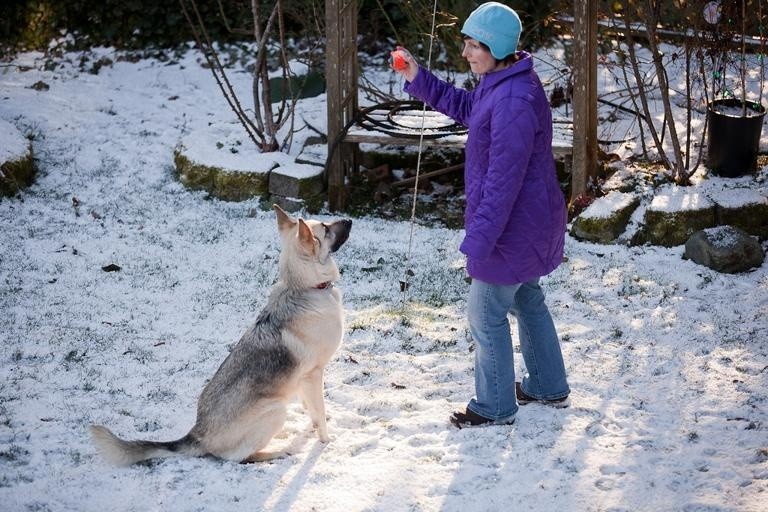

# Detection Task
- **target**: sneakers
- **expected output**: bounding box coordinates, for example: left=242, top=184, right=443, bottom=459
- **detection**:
left=448, top=407, right=516, bottom=431
left=513, top=380, right=571, bottom=411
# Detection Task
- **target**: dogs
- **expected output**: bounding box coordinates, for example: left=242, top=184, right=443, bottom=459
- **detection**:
left=85, top=203, right=353, bottom=469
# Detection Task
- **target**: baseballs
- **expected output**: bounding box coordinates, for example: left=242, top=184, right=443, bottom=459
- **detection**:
left=389, top=50, right=410, bottom=72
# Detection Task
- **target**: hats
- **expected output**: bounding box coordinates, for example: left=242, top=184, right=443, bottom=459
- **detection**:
left=457, top=1, right=523, bottom=61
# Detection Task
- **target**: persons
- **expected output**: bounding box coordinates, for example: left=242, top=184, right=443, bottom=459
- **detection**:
left=389, top=2, right=573, bottom=429
